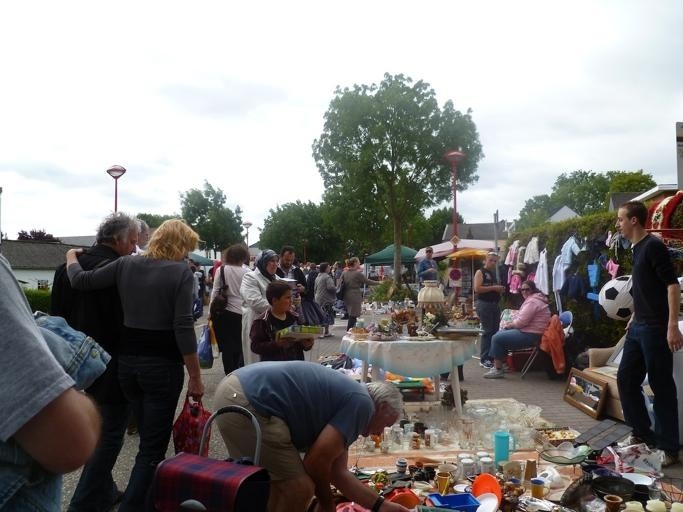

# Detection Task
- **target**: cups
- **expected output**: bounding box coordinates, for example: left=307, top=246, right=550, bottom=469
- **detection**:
left=366, top=441, right=376, bottom=452
left=508, top=479, right=526, bottom=494
left=495, top=431, right=516, bottom=470
left=461, top=458, right=478, bottom=480
left=435, top=472, right=451, bottom=495
left=384, top=427, right=397, bottom=448
left=394, top=428, right=403, bottom=445
left=480, top=457, right=496, bottom=474
left=425, top=430, right=437, bottom=449
left=455, top=418, right=478, bottom=450
left=438, top=464, right=457, bottom=485
left=404, top=424, right=415, bottom=435
left=352, top=327, right=364, bottom=341
left=531, top=480, right=550, bottom=500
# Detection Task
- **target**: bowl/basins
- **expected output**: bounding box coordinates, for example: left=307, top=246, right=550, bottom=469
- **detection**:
left=453, top=484, right=469, bottom=494
left=592, top=476, right=635, bottom=502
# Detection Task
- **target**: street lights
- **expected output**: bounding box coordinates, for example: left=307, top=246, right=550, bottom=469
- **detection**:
left=242, top=220, right=252, bottom=248
left=445, top=151, right=466, bottom=304
left=106, top=165, right=126, bottom=214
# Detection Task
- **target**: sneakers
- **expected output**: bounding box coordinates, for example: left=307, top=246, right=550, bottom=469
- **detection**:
left=483, top=368, right=505, bottom=379
left=652, top=450, right=673, bottom=466
left=618, top=436, right=645, bottom=448
left=490, top=362, right=512, bottom=373
left=479, top=360, right=492, bottom=368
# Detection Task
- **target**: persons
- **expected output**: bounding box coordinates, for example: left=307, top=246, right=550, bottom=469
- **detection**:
left=239, top=249, right=292, bottom=367
left=484, top=280, right=550, bottom=379
left=314, top=262, right=340, bottom=339
left=0, top=252, right=111, bottom=511
left=185, top=256, right=203, bottom=273
left=67, top=219, right=204, bottom=512
left=276, top=246, right=308, bottom=302
left=130, top=218, right=151, bottom=256
left=190, top=266, right=203, bottom=302
left=306, top=263, right=318, bottom=300
left=366, top=264, right=386, bottom=280
left=208, top=243, right=254, bottom=375
left=340, top=257, right=385, bottom=332
left=249, top=280, right=314, bottom=361
left=615, top=201, right=682, bottom=466
left=214, top=360, right=413, bottom=512
left=472, top=251, right=506, bottom=368
left=418, top=248, right=439, bottom=324
left=50, top=212, right=138, bottom=512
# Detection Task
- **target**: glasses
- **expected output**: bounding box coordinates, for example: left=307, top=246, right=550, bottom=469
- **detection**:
left=426, top=250, right=432, bottom=253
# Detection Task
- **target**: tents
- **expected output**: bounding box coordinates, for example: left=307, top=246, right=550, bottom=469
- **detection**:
left=364, top=243, right=419, bottom=264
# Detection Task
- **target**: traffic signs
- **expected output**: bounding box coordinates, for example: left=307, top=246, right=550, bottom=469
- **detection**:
left=448, top=267, right=463, bottom=288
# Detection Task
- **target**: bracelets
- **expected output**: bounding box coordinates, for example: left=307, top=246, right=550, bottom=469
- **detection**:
left=372, top=496, right=385, bottom=512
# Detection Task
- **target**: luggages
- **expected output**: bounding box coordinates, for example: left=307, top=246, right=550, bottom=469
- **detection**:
left=152, top=405, right=271, bottom=512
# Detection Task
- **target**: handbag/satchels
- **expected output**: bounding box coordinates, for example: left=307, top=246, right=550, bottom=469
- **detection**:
left=210, top=266, right=227, bottom=316
left=337, top=274, right=345, bottom=299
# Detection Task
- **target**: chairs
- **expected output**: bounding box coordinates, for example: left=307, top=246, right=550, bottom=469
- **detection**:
left=520, top=311, right=573, bottom=379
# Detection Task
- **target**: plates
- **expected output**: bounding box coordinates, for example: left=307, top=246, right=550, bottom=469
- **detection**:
left=472, top=473, right=502, bottom=509
left=536, top=442, right=593, bottom=464
left=619, top=473, right=653, bottom=486
left=475, top=493, right=499, bottom=512
